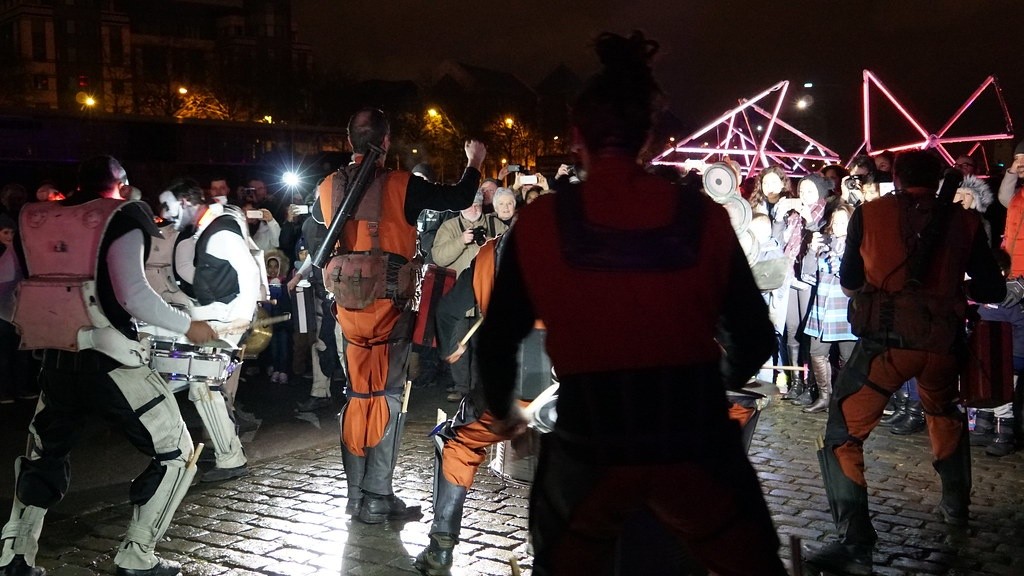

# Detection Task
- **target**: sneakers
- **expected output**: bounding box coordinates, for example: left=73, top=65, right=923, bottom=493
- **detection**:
left=938, top=496, right=970, bottom=526
left=414, top=544, right=453, bottom=576
left=359, top=494, right=421, bottom=523
left=299, top=395, right=331, bottom=412
left=344, top=497, right=363, bottom=516
left=801, top=539, right=873, bottom=576
left=115, top=556, right=182, bottom=576
left=201, top=463, right=248, bottom=482
left=0, top=558, right=46, bottom=576
left=448, top=391, right=464, bottom=401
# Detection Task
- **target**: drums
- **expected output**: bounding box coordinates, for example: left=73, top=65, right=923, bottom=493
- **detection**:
left=959, top=316, right=1017, bottom=411
left=146, top=337, right=233, bottom=389
left=701, top=161, right=762, bottom=269
left=411, top=264, right=457, bottom=349
left=492, top=418, right=537, bottom=490
left=534, top=394, right=558, bottom=431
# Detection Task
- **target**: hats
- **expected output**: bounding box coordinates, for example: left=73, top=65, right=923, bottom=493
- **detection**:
left=411, top=163, right=434, bottom=182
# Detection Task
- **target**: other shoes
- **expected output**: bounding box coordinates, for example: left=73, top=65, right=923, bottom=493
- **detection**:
left=333, top=369, right=345, bottom=381
left=235, top=412, right=258, bottom=433
left=244, top=367, right=258, bottom=377
left=279, top=373, right=288, bottom=385
left=304, top=371, right=313, bottom=379
left=266, top=367, right=275, bottom=376
left=271, top=371, right=280, bottom=383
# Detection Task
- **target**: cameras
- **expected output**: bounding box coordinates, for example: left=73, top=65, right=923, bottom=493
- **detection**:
left=243, top=188, right=256, bottom=199
left=469, top=226, right=487, bottom=247
left=566, top=165, right=576, bottom=174
left=846, top=174, right=867, bottom=190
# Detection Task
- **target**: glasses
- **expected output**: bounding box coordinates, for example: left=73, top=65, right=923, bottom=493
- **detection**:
left=953, top=163, right=974, bottom=169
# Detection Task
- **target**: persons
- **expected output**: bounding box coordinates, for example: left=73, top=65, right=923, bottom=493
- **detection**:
left=663, top=151, right=897, bottom=416
left=0, top=153, right=218, bottom=575
left=311, top=110, right=487, bottom=524
left=803, top=149, right=1008, bottom=576
left=0, top=177, right=65, bottom=404
left=414, top=230, right=556, bottom=576
left=475, top=29, right=790, bottom=576
left=411, top=162, right=578, bottom=402
left=144, top=176, right=345, bottom=483
left=879, top=141, right=1024, bottom=457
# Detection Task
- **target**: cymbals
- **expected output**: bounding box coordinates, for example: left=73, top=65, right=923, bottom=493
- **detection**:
left=741, top=378, right=781, bottom=396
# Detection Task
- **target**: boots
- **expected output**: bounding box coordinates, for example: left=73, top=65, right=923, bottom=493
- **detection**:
left=780, top=344, right=804, bottom=399
left=986, top=416, right=1017, bottom=457
left=878, top=397, right=908, bottom=426
left=891, top=401, right=925, bottom=435
left=803, top=354, right=833, bottom=414
left=968, top=410, right=995, bottom=447
left=792, top=358, right=819, bottom=405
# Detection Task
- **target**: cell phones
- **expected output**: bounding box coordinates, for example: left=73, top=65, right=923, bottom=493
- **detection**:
left=520, top=176, right=537, bottom=184
left=780, top=198, right=802, bottom=212
left=507, top=165, right=521, bottom=172
left=294, top=205, right=308, bottom=214
left=247, top=211, right=263, bottom=218
left=818, top=233, right=832, bottom=245
